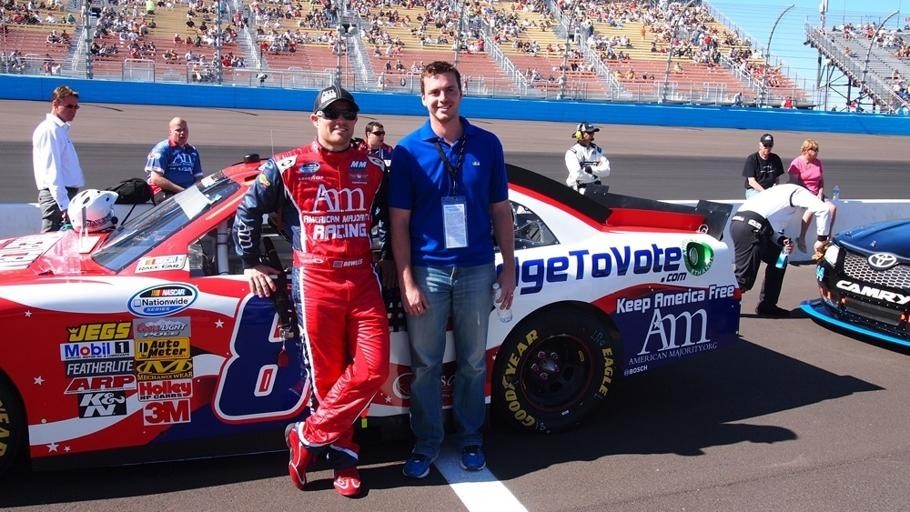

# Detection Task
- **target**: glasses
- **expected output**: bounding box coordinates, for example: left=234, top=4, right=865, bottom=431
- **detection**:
left=317, top=109, right=356, bottom=120
left=369, top=131, right=385, bottom=135
left=58, top=101, right=79, bottom=109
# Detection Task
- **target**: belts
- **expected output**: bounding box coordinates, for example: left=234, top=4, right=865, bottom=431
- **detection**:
left=731, top=215, right=761, bottom=229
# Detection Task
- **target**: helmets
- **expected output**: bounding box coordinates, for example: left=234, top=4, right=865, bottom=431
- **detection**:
left=575, top=122, right=600, bottom=140
left=68, top=189, right=118, bottom=233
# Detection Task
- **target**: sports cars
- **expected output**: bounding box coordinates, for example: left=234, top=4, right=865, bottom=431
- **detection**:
left=1, top=149, right=742, bottom=495
left=799, top=218, right=910, bottom=348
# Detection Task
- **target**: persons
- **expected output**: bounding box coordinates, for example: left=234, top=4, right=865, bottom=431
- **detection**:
left=359, top=121, right=393, bottom=160
left=742, top=133, right=784, bottom=200
left=787, top=139, right=837, bottom=254
left=386, top=61, right=516, bottom=479
left=144, top=117, right=205, bottom=203
left=32, top=86, right=86, bottom=233
left=231, top=84, right=397, bottom=496
left=1, top=0, right=909, bottom=117
left=565, top=122, right=610, bottom=195
left=729, top=179, right=831, bottom=318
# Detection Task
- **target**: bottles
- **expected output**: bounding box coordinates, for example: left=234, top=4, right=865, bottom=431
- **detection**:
left=775, top=249, right=791, bottom=269
left=832, top=184, right=840, bottom=201
left=490, top=280, right=513, bottom=323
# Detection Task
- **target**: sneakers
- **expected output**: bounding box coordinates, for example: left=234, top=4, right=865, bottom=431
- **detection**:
left=285, top=423, right=312, bottom=489
left=403, top=451, right=439, bottom=477
left=459, top=444, right=486, bottom=471
left=334, top=464, right=363, bottom=495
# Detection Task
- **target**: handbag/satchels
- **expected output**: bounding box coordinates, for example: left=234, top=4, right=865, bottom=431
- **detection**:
left=106, top=178, right=155, bottom=204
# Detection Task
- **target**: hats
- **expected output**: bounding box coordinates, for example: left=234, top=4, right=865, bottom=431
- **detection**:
left=314, top=87, right=359, bottom=114
left=761, top=134, right=773, bottom=146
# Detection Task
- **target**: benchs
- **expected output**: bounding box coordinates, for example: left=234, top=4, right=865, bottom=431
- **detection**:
left=1, top=0, right=814, bottom=107
left=804, top=24, right=910, bottom=114
